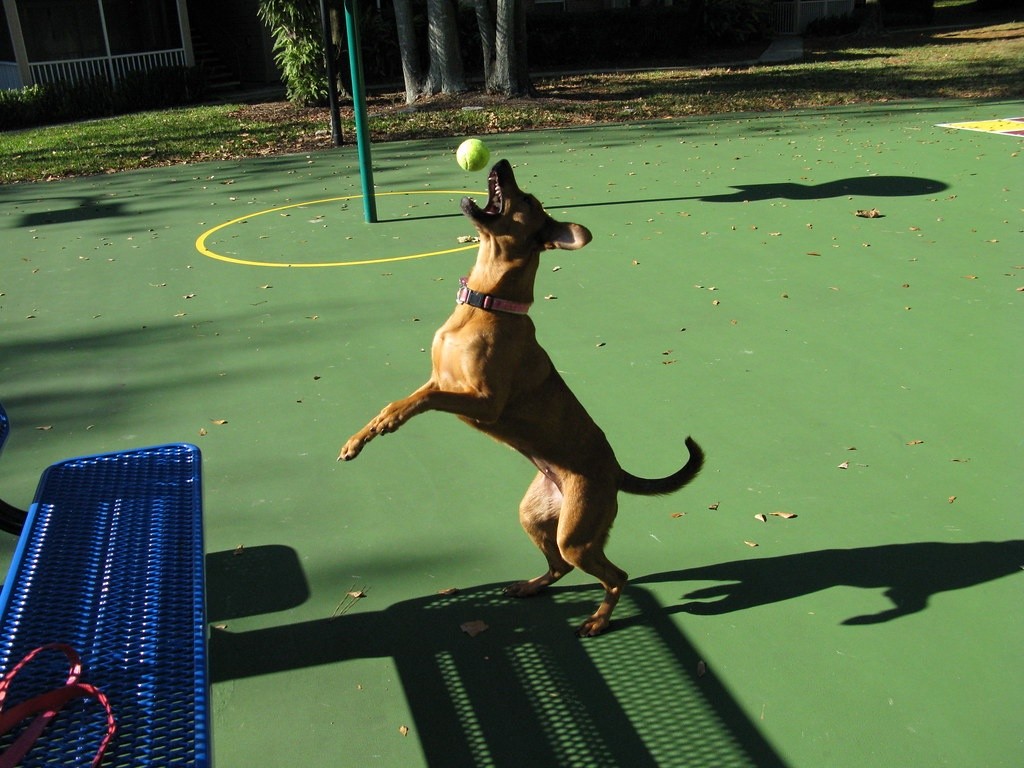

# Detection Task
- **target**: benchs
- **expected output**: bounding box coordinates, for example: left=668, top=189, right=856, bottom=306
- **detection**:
left=0, top=406, right=212, bottom=768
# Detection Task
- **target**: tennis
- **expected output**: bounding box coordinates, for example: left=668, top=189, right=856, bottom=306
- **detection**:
left=456, top=137, right=490, bottom=172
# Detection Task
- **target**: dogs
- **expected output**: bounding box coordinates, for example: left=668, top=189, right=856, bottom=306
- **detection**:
left=337, top=157, right=706, bottom=638
